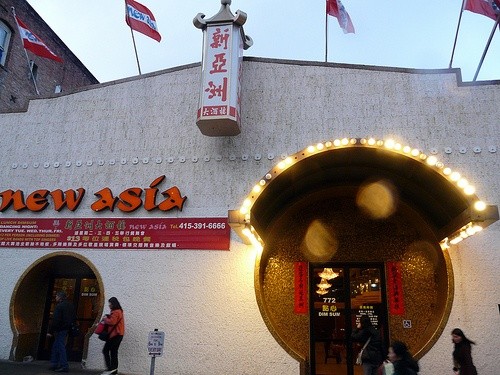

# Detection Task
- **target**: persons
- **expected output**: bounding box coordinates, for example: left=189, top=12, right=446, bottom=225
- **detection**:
left=101, top=297, right=124, bottom=375
left=387, top=341, right=419, bottom=375
left=351, top=315, right=383, bottom=375
left=450, top=328, right=477, bottom=375
left=46, top=290, right=72, bottom=372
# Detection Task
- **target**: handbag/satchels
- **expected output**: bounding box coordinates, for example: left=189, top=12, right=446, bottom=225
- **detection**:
left=98, top=326, right=109, bottom=341
left=355, top=349, right=364, bottom=366
left=69, top=320, right=80, bottom=337
left=382, top=360, right=395, bottom=375
left=95, top=321, right=105, bottom=335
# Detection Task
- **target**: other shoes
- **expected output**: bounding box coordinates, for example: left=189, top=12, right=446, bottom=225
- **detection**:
left=100, top=368, right=118, bottom=375
left=48, top=363, right=69, bottom=373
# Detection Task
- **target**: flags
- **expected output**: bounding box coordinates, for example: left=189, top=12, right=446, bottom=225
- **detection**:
left=124, top=0, right=161, bottom=43
left=326, top=0, right=356, bottom=34
left=14, top=14, right=64, bottom=62
left=463, top=0, right=500, bottom=30
